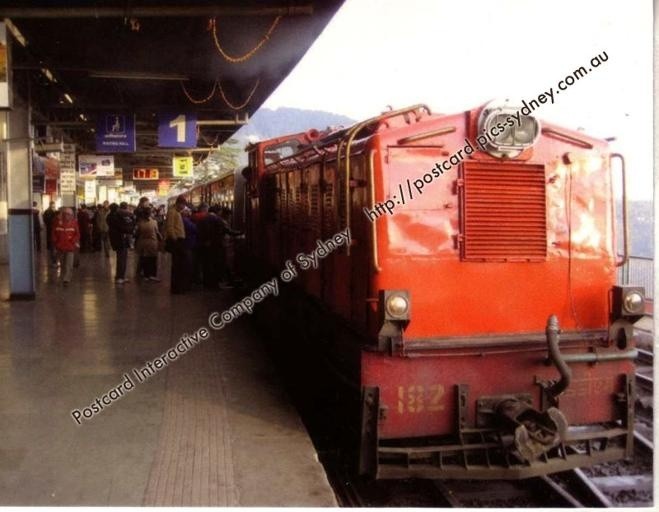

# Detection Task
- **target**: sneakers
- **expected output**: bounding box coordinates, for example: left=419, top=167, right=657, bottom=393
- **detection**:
left=124, top=277, right=131, bottom=282
left=115, top=279, right=123, bottom=285
left=144, top=276, right=161, bottom=282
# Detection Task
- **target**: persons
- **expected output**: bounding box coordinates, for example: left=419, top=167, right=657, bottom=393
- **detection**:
left=242, top=166, right=252, bottom=180
left=33, top=196, right=243, bottom=294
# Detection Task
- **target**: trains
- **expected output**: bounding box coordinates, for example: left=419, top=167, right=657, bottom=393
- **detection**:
left=166, top=94, right=646, bottom=483
